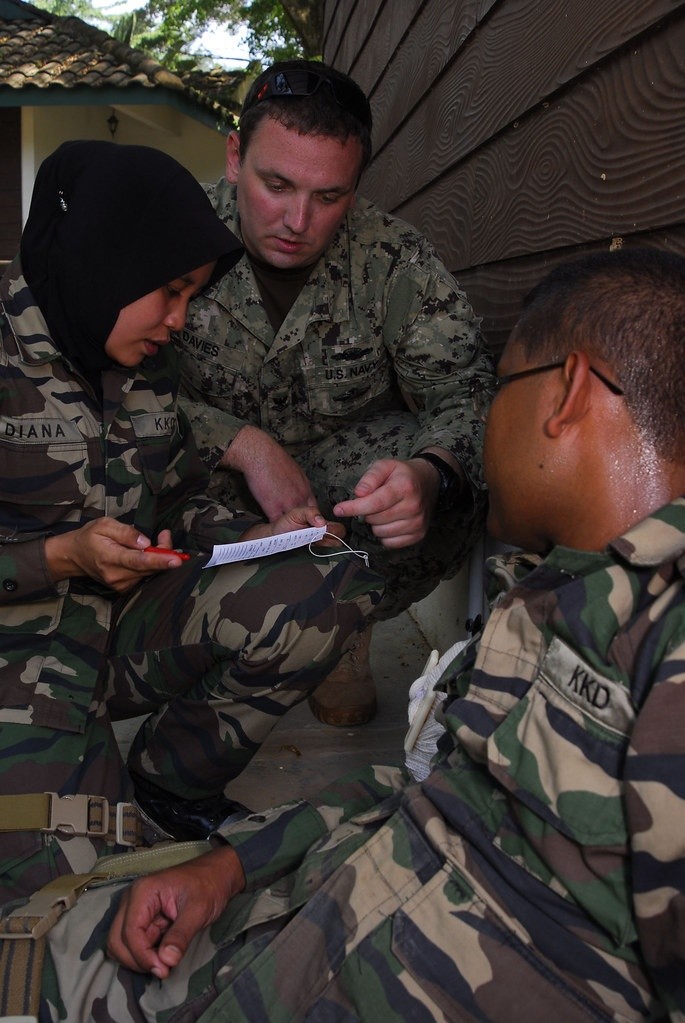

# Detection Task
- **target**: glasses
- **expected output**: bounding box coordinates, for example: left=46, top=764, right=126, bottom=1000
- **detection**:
left=468, top=361, right=624, bottom=424
left=239, top=68, right=372, bottom=131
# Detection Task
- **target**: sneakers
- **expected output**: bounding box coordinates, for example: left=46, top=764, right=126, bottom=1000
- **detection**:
left=308, top=622, right=379, bottom=725
left=125, top=764, right=259, bottom=843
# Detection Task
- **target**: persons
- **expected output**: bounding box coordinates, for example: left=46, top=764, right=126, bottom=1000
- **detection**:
left=130, top=59, right=505, bottom=628
left=2, top=131, right=380, bottom=899
left=29, top=249, right=684, bottom=1023
